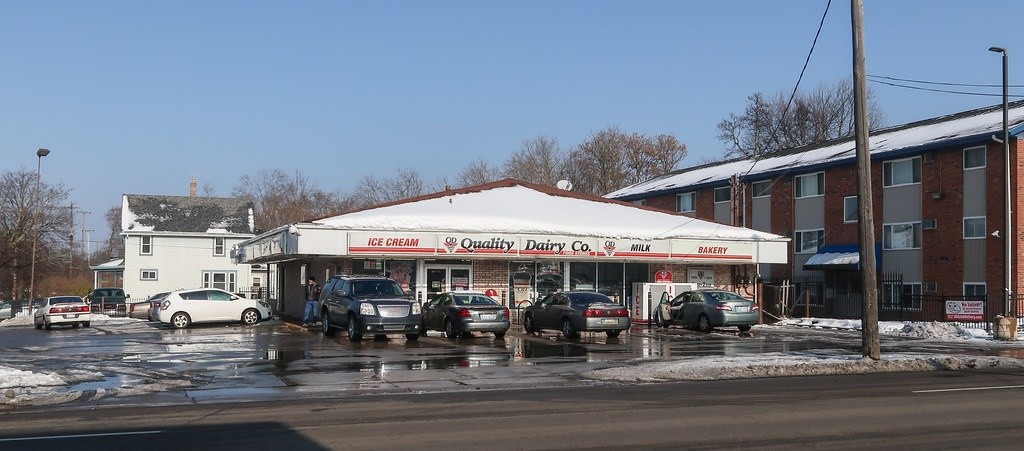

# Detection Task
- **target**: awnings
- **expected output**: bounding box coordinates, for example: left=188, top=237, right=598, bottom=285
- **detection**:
left=803, top=243, right=882, bottom=273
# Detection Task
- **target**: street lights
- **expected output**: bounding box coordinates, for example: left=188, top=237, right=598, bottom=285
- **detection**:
left=988, top=45, right=1017, bottom=342
left=28, top=148, right=51, bottom=315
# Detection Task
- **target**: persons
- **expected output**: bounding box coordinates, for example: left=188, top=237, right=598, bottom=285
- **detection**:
left=303, top=276, right=321, bottom=327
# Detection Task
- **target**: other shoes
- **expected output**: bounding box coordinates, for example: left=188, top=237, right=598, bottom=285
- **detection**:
left=302, top=324, right=308, bottom=327
left=312, top=323, right=316, bottom=327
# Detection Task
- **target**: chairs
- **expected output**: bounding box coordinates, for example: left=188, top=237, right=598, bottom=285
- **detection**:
left=456, top=298, right=464, bottom=305
left=472, top=300, right=480, bottom=304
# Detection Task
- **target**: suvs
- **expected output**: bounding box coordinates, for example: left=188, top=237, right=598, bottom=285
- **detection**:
left=89, top=288, right=130, bottom=316
left=318, top=273, right=424, bottom=341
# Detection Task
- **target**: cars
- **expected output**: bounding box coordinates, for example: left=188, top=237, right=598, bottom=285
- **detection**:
left=129, top=293, right=157, bottom=322
left=652, top=288, right=759, bottom=333
left=148, top=289, right=274, bottom=328
left=33, top=296, right=91, bottom=330
left=0, top=298, right=44, bottom=319
left=420, top=292, right=510, bottom=339
left=520, top=291, right=632, bottom=338
